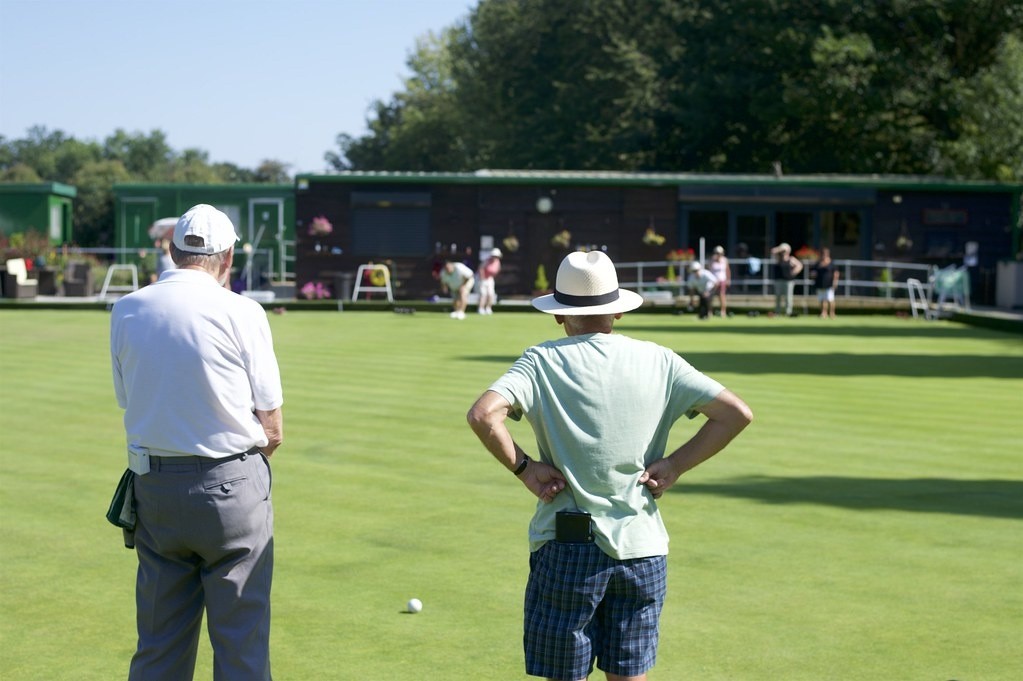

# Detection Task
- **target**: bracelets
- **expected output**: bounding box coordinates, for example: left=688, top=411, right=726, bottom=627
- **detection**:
left=512, top=453, right=530, bottom=475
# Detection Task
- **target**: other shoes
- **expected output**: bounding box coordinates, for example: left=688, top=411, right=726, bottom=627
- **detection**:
left=478, top=308, right=495, bottom=314
left=450, top=310, right=466, bottom=320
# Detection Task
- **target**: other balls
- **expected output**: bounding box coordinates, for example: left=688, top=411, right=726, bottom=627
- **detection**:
left=408, top=598, right=422, bottom=614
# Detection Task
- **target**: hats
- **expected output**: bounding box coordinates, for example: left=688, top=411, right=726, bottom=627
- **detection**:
left=491, top=248, right=502, bottom=257
left=714, top=245, right=725, bottom=254
left=691, top=261, right=701, bottom=272
left=172, top=204, right=241, bottom=256
left=532, top=250, right=643, bottom=315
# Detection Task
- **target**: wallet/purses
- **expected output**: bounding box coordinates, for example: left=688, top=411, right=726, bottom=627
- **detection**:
left=555, top=511, right=592, bottom=543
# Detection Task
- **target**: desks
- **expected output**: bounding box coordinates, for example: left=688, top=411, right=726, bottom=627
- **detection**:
left=39, top=271, right=59, bottom=295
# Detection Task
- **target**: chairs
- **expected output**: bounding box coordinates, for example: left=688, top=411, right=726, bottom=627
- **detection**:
left=4, top=256, right=39, bottom=300
left=61, top=259, right=92, bottom=298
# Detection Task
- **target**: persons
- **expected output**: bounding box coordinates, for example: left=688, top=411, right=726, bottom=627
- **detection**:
left=770, top=243, right=803, bottom=317
left=429, top=248, right=503, bottom=319
left=466, top=251, right=752, bottom=680
left=809, top=247, right=839, bottom=317
left=685, top=242, right=751, bottom=320
left=107, top=203, right=284, bottom=680
